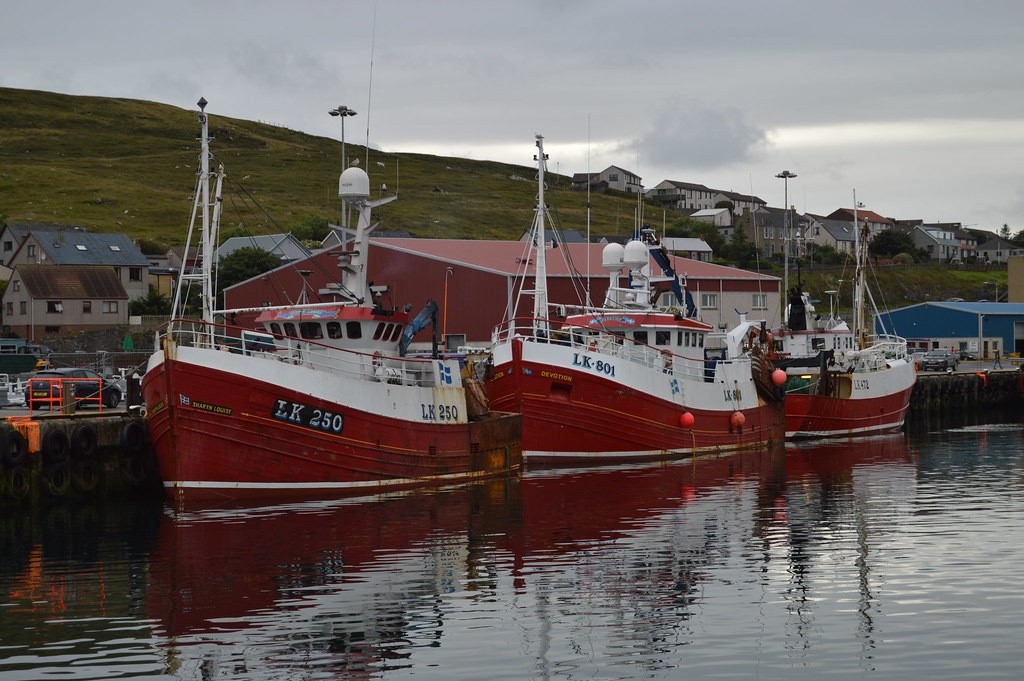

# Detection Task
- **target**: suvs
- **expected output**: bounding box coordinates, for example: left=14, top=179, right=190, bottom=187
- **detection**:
left=19, top=345, right=53, bottom=362
left=907, top=348, right=960, bottom=372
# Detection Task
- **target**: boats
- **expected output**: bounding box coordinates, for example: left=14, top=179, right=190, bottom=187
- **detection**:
left=760, top=188, right=919, bottom=440
left=140, top=97, right=522, bottom=512
left=491, top=448, right=776, bottom=589
left=137, top=486, right=472, bottom=676
left=483, top=133, right=789, bottom=473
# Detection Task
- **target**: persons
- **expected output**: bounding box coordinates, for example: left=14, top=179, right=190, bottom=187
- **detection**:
left=993, top=349, right=1003, bottom=369
left=921, top=349, right=927, bottom=370
left=952, top=347, right=960, bottom=364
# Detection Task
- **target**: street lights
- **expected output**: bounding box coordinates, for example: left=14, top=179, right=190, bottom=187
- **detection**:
left=328, top=105, right=359, bottom=288
left=775, top=170, right=798, bottom=330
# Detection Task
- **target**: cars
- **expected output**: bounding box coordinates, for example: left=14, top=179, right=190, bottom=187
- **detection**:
left=960, top=352, right=977, bottom=361
left=24, top=368, right=121, bottom=410
